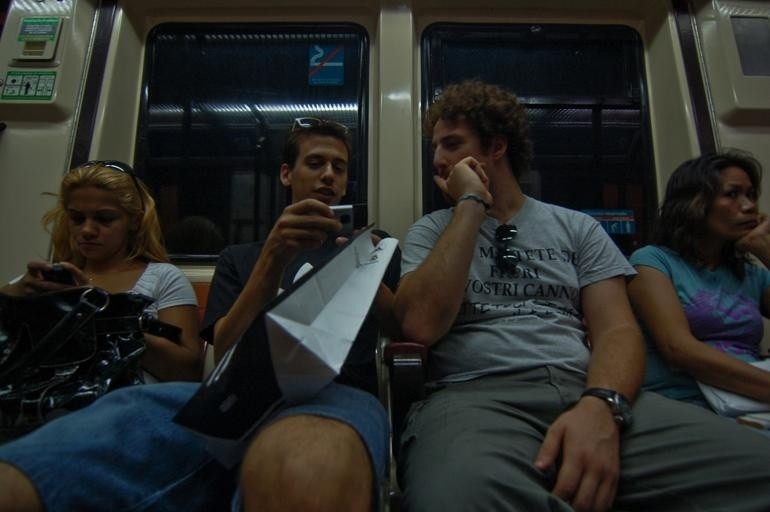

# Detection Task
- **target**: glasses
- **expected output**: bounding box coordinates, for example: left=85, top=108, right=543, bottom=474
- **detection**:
left=75, top=159, right=146, bottom=214
left=494, top=222, right=520, bottom=278
left=292, top=116, right=348, bottom=137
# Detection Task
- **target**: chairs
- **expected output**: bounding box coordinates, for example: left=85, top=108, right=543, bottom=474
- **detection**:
left=137, top=274, right=426, bottom=511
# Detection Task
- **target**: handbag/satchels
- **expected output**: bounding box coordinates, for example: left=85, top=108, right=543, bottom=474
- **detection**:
left=172, top=224, right=400, bottom=449
left=0, top=283, right=156, bottom=446
left=694, top=358, right=770, bottom=418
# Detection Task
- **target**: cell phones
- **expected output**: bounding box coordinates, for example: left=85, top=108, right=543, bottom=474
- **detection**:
left=329, top=205, right=354, bottom=233
left=41, top=264, right=79, bottom=286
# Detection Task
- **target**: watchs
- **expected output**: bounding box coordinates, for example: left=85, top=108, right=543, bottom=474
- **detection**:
left=580, top=387, right=633, bottom=430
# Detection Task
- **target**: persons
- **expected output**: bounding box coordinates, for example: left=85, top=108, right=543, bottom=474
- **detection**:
left=393, top=79, right=769, bottom=512
left=0, top=116, right=403, bottom=512
left=626, top=146, right=770, bottom=420
left=0, top=159, right=203, bottom=439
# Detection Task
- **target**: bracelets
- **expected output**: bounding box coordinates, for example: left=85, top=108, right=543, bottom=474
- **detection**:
left=457, top=193, right=490, bottom=212
